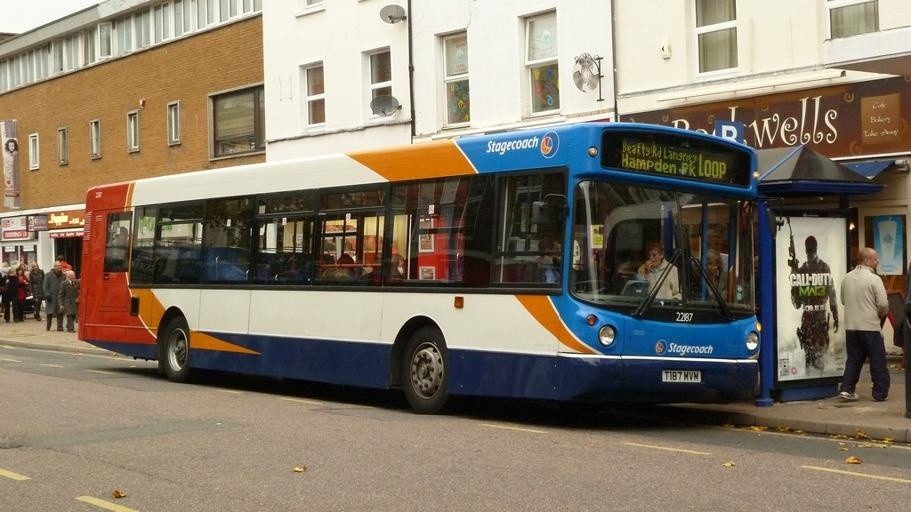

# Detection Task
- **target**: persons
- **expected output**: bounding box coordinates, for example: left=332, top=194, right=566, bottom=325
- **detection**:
left=532, top=236, right=564, bottom=284
left=4, top=139, right=18, bottom=155
left=0, top=254, right=80, bottom=333
left=637, top=239, right=681, bottom=301
left=111, top=227, right=128, bottom=258
left=841, top=247, right=891, bottom=402
left=788, top=235, right=839, bottom=378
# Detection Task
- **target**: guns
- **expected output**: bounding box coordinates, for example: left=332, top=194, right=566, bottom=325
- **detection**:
left=783, top=217, right=800, bottom=280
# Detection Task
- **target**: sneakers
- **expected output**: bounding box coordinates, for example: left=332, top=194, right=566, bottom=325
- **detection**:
left=839, top=390, right=862, bottom=402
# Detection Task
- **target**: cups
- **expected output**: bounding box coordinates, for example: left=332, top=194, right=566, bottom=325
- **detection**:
left=878, top=218, right=897, bottom=269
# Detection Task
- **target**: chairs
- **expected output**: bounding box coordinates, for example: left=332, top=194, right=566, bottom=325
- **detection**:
left=166, top=244, right=335, bottom=285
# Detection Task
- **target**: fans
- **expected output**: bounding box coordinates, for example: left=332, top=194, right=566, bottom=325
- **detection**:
left=573, top=54, right=604, bottom=101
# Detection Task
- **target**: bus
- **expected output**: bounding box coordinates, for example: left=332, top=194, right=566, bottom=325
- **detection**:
left=76, top=121, right=765, bottom=414
